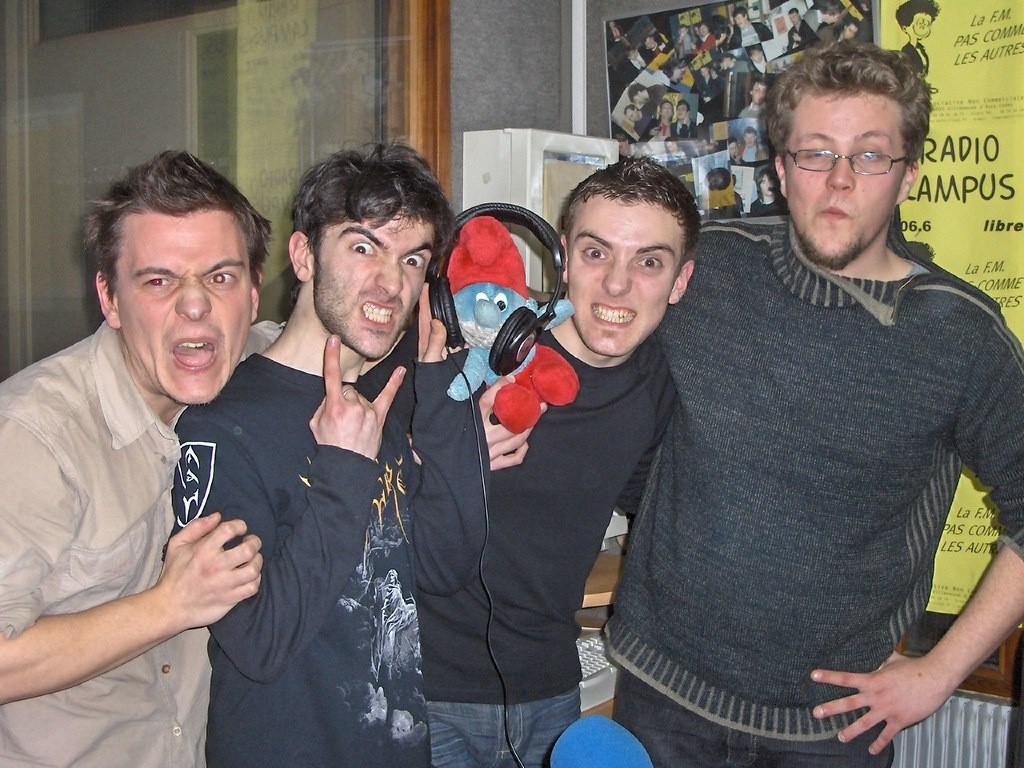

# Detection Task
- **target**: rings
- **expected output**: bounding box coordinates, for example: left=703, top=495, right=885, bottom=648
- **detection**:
left=343, top=388, right=358, bottom=398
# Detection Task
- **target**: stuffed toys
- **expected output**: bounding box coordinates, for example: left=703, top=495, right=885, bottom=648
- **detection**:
left=442, top=217, right=581, bottom=433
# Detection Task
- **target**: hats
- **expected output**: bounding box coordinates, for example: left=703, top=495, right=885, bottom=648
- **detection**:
left=754, top=164, right=776, bottom=180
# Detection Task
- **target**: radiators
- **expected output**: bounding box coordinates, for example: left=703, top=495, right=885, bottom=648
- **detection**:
left=891, top=688, right=1024, bottom=768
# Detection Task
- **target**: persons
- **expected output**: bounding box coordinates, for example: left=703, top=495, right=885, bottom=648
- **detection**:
left=1, top=154, right=287, bottom=768
left=604, top=47, right=1024, bottom=766
left=606, top=0, right=869, bottom=216
left=419, top=160, right=701, bottom=767
left=163, top=151, right=489, bottom=767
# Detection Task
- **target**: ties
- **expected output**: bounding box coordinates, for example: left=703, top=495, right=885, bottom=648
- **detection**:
left=662, top=123, right=668, bottom=135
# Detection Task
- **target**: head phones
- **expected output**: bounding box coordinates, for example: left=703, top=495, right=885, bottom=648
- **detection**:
left=428, top=201, right=565, bottom=377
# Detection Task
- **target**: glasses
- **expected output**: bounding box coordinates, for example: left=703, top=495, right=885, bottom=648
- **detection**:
left=786, top=146, right=908, bottom=176
